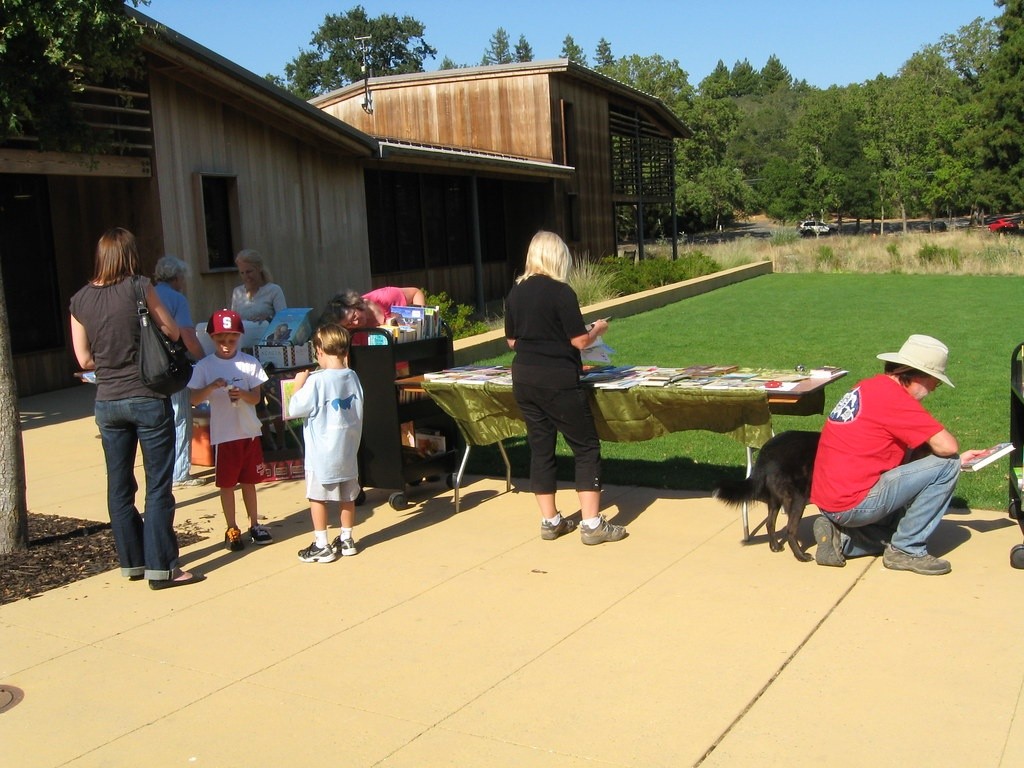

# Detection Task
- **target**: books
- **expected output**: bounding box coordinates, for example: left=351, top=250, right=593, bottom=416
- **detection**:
left=581, top=364, right=812, bottom=391
left=960, top=441, right=1016, bottom=473
left=396, top=359, right=448, bottom=458
left=281, top=379, right=304, bottom=421
left=368, top=304, right=440, bottom=346
left=424, top=363, right=513, bottom=387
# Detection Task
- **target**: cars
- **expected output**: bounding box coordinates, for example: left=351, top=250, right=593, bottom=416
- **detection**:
left=798, top=220, right=838, bottom=237
left=984, top=212, right=1024, bottom=234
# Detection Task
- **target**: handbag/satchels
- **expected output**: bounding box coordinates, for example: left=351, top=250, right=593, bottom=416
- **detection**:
left=132, top=274, right=195, bottom=395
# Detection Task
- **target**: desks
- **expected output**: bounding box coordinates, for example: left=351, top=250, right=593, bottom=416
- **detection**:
left=255, top=363, right=319, bottom=456
left=421, top=366, right=849, bottom=545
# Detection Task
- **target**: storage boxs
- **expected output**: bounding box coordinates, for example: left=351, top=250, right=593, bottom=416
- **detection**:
left=191, top=307, right=315, bottom=481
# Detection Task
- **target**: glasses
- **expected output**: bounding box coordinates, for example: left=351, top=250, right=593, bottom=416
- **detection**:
left=344, top=310, right=357, bottom=329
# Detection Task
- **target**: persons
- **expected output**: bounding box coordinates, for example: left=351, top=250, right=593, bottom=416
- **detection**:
left=503, top=231, right=628, bottom=546
left=319, top=285, right=425, bottom=346
left=69, top=226, right=206, bottom=591
left=186, top=307, right=273, bottom=551
left=809, top=334, right=987, bottom=574
left=288, top=322, right=365, bottom=563
left=147, top=254, right=206, bottom=488
left=230, top=250, right=290, bottom=321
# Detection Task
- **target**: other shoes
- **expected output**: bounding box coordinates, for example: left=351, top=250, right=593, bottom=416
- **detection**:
left=172, top=478, right=207, bottom=490
left=148, top=571, right=203, bottom=589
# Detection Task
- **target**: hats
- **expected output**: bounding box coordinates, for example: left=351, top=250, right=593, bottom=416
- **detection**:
left=206, top=307, right=246, bottom=337
left=875, top=334, right=955, bottom=391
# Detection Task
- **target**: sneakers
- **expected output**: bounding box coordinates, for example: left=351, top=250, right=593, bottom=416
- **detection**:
left=329, top=536, right=358, bottom=555
left=297, top=542, right=336, bottom=563
left=580, top=515, right=626, bottom=545
left=882, top=544, right=952, bottom=575
left=812, top=516, right=848, bottom=567
left=251, top=525, right=274, bottom=545
left=223, top=529, right=245, bottom=551
left=541, top=516, right=575, bottom=541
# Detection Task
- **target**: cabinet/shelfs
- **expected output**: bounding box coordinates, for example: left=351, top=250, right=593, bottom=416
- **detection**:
left=1008, top=343, right=1024, bottom=568
left=348, top=323, right=464, bottom=511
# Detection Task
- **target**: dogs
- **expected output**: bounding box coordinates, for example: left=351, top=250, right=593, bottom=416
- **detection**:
left=717, top=430, right=932, bottom=563
